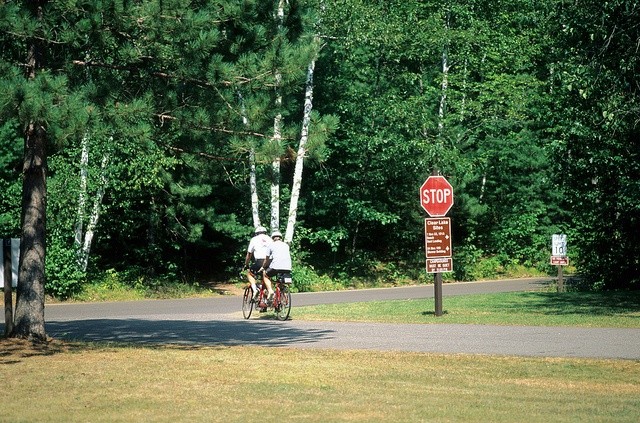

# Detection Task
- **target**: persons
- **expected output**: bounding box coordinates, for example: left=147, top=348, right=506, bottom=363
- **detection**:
left=257, top=230, right=293, bottom=306
left=242, top=226, right=273, bottom=300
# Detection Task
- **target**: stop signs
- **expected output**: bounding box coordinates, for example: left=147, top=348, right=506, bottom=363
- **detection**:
left=420, top=175, right=454, bottom=217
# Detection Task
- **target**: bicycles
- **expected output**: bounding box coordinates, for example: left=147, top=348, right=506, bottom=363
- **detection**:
left=242, top=262, right=291, bottom=321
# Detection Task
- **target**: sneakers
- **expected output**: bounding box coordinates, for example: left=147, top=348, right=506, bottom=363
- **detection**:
left=252, top=290, right=258, bottom=299
left=267, top=290, right=274, bottom=300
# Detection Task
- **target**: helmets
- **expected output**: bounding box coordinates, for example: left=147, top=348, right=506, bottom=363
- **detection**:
left=255, top=226, right=267, bottom=234
left=271, top=232, right=282, bottom=239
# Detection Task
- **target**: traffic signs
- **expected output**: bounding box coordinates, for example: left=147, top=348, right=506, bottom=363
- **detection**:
left=552, top=234, right=567, bottom=255
left=425, top=217, right=452, bottom=258
left=426, top=257, right=453, bottom=273
left=550, top=256, right=569, bottom=265
